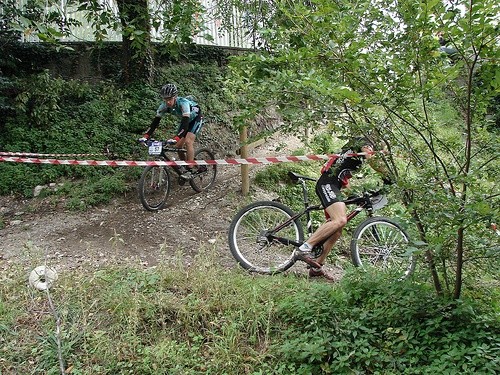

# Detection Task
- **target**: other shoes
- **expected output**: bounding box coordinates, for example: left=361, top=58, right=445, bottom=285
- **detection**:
left=180, top=172, right=193, bottom=179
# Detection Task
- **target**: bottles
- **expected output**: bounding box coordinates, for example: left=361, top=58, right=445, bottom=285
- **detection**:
left=171, top=157, right=184, bottom=171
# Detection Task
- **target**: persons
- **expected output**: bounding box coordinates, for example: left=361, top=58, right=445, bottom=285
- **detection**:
left=295, top=130, right=397, bottom=282
left=142, top=84, right=204, bottom=178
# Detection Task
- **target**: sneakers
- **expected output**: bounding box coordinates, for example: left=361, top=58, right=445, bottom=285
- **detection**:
left=294, top=247, right=321, bottom=269
left=309, top=268, right=334, bottom=280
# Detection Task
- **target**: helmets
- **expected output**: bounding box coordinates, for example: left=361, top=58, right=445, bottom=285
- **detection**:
left=160, top=84, right=177, bottom=98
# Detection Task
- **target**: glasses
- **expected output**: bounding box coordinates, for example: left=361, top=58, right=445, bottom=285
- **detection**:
left=162, top=96, right=174, bottom=102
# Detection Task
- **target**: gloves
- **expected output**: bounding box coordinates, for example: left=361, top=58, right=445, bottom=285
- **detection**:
left=167, top=139, right=177, bottom=145
left=138, top=137, right=148, bottom=143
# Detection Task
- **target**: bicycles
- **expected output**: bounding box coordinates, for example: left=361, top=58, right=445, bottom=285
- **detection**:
left=133, top=134, right=218, bottom=211
left=226, top=171, right=417, bottom=283
